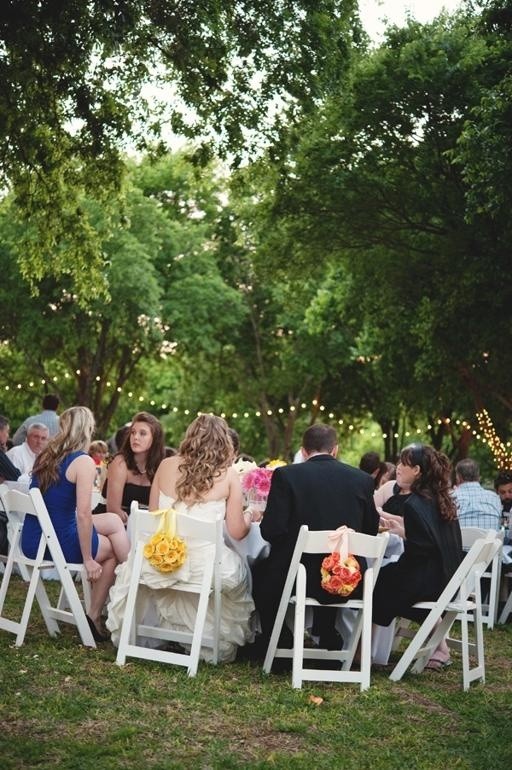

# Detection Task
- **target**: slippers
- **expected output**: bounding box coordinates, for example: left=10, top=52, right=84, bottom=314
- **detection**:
left=424, top=655, right=454, bottom=671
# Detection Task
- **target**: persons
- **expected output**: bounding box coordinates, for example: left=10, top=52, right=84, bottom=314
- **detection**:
left=1, top=395, right=511, bottom=673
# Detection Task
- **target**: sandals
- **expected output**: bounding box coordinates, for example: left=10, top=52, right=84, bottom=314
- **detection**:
left=85, top=613, right=108, bottom=641
